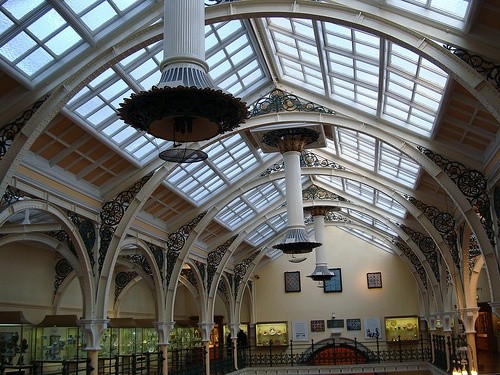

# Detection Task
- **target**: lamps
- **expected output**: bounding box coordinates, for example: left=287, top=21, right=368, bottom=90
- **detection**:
left=260, top=128, right=324, bottom=264
left=303, top=206, right=337, bottom=281
left=116, top=0, right=251, bottom=164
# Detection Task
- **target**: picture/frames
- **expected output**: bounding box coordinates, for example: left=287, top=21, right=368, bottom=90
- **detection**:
left=284, top=271, right=301, bottom=293
left=291, top=320, right=309, bottom=342
left=323, top=268, right=342, bottom=293
left=367, top=272, right=382, bottom=289
left=363, top=315, right=382, bottom=339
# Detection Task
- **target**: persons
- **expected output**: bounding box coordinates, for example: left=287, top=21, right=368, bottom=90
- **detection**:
left=226, top=329, right=249, bottom=362
